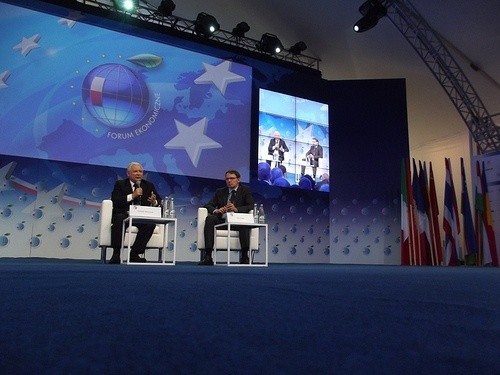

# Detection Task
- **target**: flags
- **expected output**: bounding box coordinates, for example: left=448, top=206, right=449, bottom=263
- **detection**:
left=401, top=158, right=500, bottom=267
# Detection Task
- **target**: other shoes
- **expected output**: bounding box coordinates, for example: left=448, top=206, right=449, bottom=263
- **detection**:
left=198, top=257, right=213, bottom=266
left=241, top=258, right=253, bottom=264
left=129, top=255, right=146, bottom=263
left=108, top=255, right=120, bottom=265
left=313, top=175, right=316, bottom=178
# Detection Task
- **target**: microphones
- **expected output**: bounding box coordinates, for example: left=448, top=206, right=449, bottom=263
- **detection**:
left=136, top=181, right=141, bottom=198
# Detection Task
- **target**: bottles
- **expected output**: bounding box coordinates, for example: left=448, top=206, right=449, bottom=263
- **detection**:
left=162, top=197, right=169, bottom=218
left=253, top=203, right=259, bottom=224
left=259, top=205, right=265, bottom=224
left=169, top=197, right=175, bottom=218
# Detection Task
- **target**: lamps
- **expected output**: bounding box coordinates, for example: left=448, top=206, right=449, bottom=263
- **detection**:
left=194, top=12, right=220, bottom=37
left=255, top=32, right=284, bottom=56
left=158, top=0, right=176, bottom=17
left=352, top=0, right=388, bottom=33
left=231, top=22, right=250, bottom=37
left=289, top=40, right=307, bottom=55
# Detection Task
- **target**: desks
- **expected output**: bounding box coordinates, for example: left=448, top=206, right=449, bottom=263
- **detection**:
left=213, top=222, right=268, bottom=267
left=120, top=217, right=178, bottom=265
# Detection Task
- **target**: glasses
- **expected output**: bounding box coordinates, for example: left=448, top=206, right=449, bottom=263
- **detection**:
left=225, top=177, right=238, bottom=180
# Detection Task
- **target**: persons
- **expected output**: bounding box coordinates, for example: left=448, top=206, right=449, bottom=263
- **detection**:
left=301, top=138, right=323, bottom=180
left=258, top=162, right=329, bottom=192
left=266, top=132, right=289, bottom=168
left=198, top=170, right=253, bottom=265
left=108, top=162, right=162, bottom=264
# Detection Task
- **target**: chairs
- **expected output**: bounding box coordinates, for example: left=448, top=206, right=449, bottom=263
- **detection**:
left=196, top=208, right=259, bottom=264
left=98, top=200, right=169, bottom=264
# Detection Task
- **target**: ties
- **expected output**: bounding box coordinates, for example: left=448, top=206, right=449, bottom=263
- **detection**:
left=230, top=189, right=236, bottom=204
left=133, top=182, right=141, bottom=205
left=277, top=140, right=278, bottom=143
